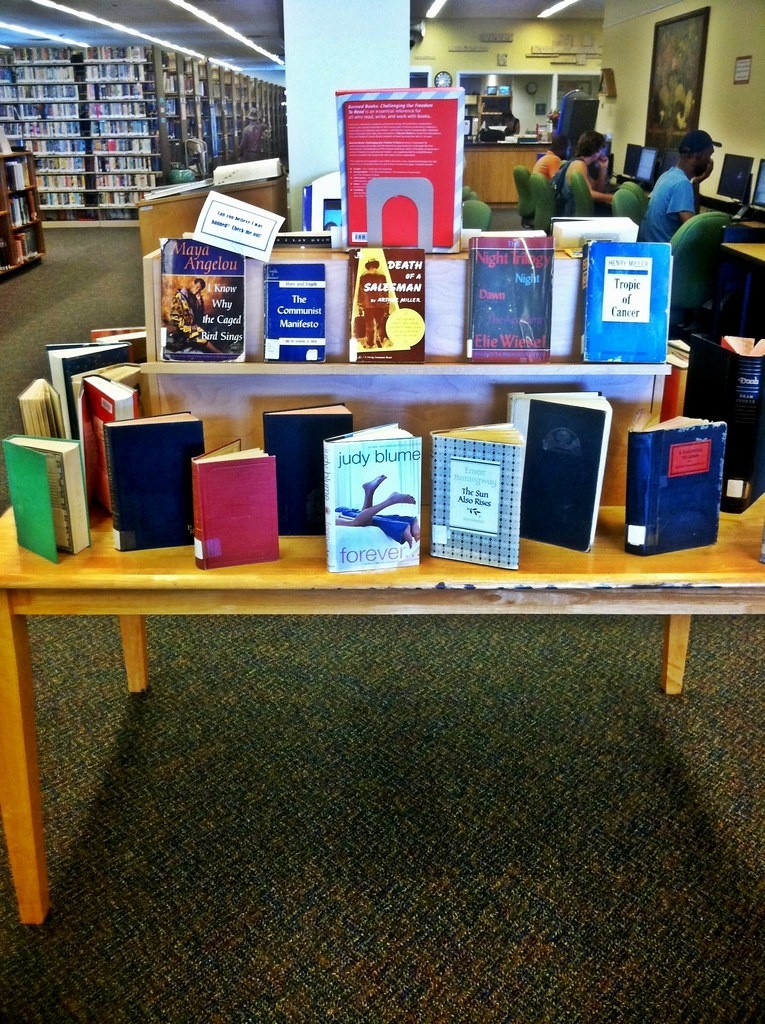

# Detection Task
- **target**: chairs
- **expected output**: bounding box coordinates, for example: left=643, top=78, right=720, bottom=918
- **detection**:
left=462, top=185, right=492, bottom=231
left=513, top=166, right=557, bottom=236
left=669, top=213, right=732, bottom=308
left=570, top=171, right=594, bottom=216
left=612, top=181, right=648, bottom=226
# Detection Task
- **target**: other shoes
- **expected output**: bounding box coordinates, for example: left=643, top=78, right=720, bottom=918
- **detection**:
left=376, top=340, right=382, bottom=348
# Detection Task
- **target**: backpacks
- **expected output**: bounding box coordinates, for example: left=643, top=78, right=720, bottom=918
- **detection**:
left=550, top=156, right=587, bottom=216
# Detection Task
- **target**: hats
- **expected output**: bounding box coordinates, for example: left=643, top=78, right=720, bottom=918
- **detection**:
left=679, top=130, right=722, bottom=153
left=365, top=259, right=379, bottom=268
left=247, top=108, right=260, bottom=120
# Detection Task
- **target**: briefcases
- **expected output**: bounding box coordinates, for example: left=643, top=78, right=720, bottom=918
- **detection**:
left=355, top=315, right=366, bottom=338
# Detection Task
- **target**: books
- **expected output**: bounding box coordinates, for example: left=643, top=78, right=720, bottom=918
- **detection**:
left=2, top=326, right=204, bottom=564
left=0, top=45, right=287, bottom=271
left=263, top=263, right=326, bottom=363
left=191, top=439, right=280, bottom=570
left=323, top=422, right=423, bottom=572
left=466, top=236, right=554, bottom=364
left=428, top=423, right=526, bottom=571
left=159, top=238, right=246, bottom=363
left=262, top=403, right=354, bottom=536
left=580, top=242, right=674, bottom=363
left=624, top=412, right=728, bottom=557
left=507, top=391, right=613, bottom=552
left=682, top=334, right=765, bottom=514
left=346, top=249, right=425, bottom=365
left=336, top=87, right=466, bottom=254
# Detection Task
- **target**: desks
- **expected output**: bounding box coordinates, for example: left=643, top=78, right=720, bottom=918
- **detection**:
left=721, top=243, right=765, bottom=337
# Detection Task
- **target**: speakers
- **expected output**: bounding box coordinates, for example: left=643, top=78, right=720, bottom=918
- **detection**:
left=558, top=90, right=600, bottom=140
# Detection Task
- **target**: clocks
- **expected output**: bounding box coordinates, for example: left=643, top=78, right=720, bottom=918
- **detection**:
left=435, top=71, right=453, bottom=87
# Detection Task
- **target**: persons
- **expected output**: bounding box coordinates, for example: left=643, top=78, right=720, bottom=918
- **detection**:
left=532, top=131, right=613, bottom=217
left=358, top=258, right=391, bottom=348
left=500, top=110, right=520, bottom=136
left=335, top=474, right=421, bottom=548
left=638, top=130, right=722, bottom=242
left=653, top=21, right=699, bottom=136
left=170, top=279, right=220, bottom=354
left=230, top=108, right=270, bottom=162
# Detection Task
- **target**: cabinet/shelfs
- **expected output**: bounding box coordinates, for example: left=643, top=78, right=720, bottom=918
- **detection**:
left=0, top=249, right=765, bottom=924
left=0, top=44, right=288, bottom=227
left=477, top=94, right=512, bottom=132
left=0, top=151, right=46, bottom=276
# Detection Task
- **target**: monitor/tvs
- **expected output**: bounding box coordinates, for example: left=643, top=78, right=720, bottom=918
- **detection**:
left=623, top=143, right=681, bottom=189
left=716, top=153, right=765, bottom=221
